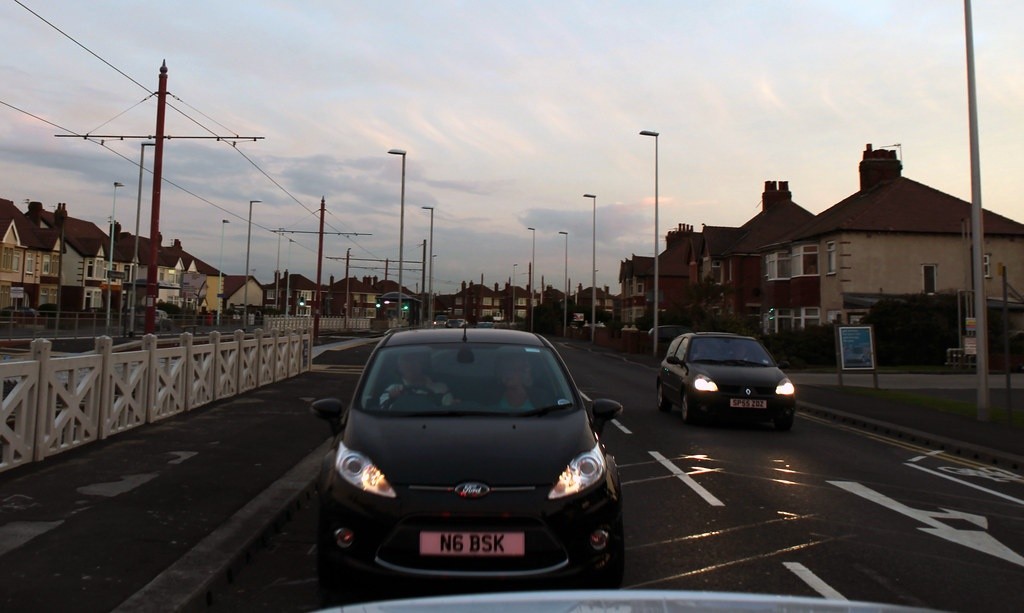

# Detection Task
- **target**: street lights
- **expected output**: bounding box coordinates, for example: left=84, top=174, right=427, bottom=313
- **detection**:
left=216, top=219, right=230, bottom=326
left=388, top=149, right=407, bottom=325
left=513, top=263, right=518, bottom=323
left=242, top=199, right=261, bottom=328
left=582, top=194, right=596, bottom=343
left=422, top=206, right=434, bottom=328
left=129, top=140, right=155, bottom=337
left=558, top=231, right=567, bottom=337
left=639, top=129, right=659, bottom=357
left=106, top=181, right=125, bottom=329
left=527, top=228, right=534, bottom=333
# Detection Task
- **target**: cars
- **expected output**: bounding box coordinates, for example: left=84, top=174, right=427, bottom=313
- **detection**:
left=433, top=314, right=506, bottom=330
left=649, top=325, right=689, bottom=341
left=309, top=325, right=625, bottom=604
left=656, top=331, right=798, bottom=433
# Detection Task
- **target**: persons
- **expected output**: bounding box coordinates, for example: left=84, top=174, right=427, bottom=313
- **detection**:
left=730, top=345, right=752, bottom=361
left=379, top=351, right=454, bottom=408
left=485, top=356, right=554, bottom=412
